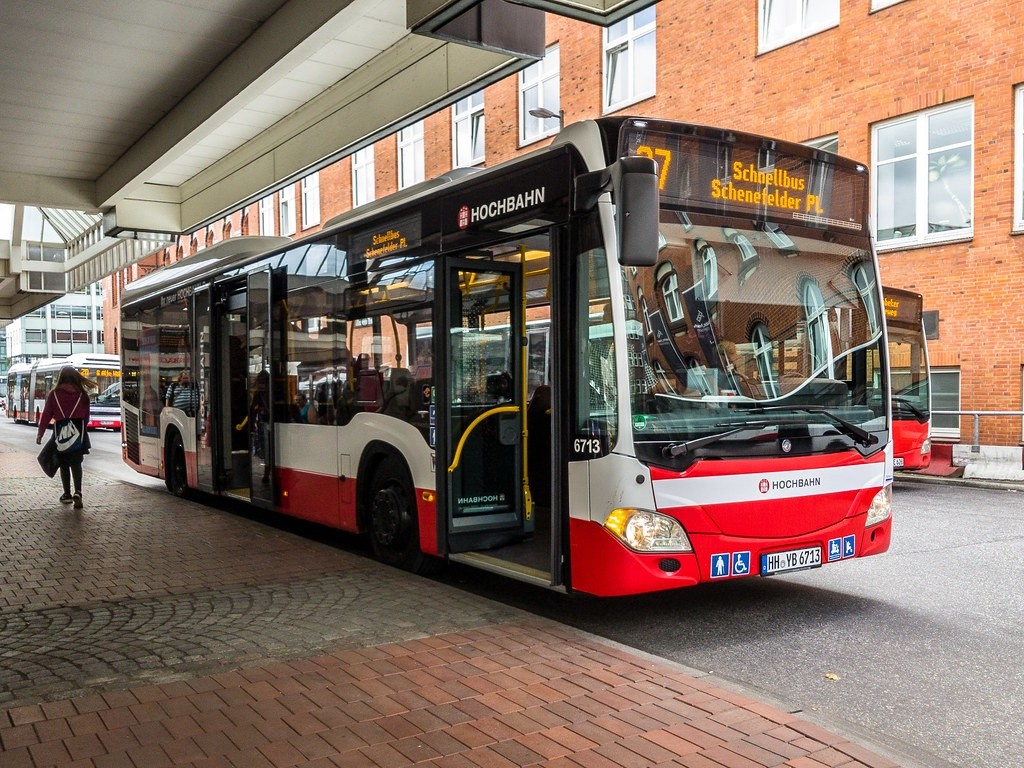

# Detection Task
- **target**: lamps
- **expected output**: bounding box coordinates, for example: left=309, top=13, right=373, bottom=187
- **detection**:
left=528, top=107, right=565, bottom=131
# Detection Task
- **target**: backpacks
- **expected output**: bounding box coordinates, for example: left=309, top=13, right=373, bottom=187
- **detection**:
left=51, top=418, right=86, bottom=452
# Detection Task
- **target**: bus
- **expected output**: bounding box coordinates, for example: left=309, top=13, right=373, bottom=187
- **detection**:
left=5, top=353, right=123, bottom=432
left=415, top=285, right=937, bottom=480
left=116, top=111, right=893, bottom=599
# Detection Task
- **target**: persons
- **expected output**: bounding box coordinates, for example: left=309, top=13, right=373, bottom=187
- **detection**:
left=717, top=340, right=743, bottom=408
left=141, top=353, right=159, bottom=427
left=587, top=302, right=666, bottom=452
left=36, top=366, right=98, bottom=509
left=246, top=369, right=416, bottom=487
left=166, top=371, right=204, bottom=417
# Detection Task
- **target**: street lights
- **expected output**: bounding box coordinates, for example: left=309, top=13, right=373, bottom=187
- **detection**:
left=58, top=311, right=73, bottom=354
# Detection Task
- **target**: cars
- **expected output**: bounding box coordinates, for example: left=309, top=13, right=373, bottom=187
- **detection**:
left=0, top=376, right=7, bottom=397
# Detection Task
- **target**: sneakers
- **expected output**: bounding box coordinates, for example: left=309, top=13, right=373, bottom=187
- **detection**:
left=60, top=490, right=83, bottom=510
